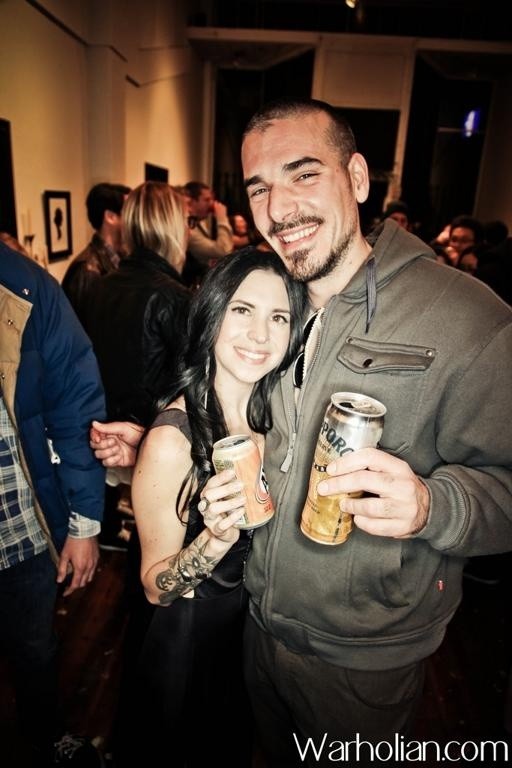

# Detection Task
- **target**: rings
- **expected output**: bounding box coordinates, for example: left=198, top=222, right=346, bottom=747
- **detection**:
left=200, top=509, right=218, bottom=521
left=196, top=496, right=212, bottom=513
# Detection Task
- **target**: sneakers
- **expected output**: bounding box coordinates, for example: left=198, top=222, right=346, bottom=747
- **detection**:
left=97, top=525, right=132, bottom=555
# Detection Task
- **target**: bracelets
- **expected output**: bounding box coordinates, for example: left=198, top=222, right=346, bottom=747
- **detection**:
left=176, top=548, right=212, bottom=583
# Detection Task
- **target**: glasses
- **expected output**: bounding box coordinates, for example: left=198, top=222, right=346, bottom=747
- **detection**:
left=291, top=304, right=325, bottom=391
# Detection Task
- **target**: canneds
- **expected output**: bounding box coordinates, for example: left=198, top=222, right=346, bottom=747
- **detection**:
left=300, top=391, right=387, bottom=545
left=212, top=435, right=275, bottom=529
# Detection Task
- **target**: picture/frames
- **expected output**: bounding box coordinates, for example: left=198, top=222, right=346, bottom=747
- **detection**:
left=42, top=189, right=74, bottom=265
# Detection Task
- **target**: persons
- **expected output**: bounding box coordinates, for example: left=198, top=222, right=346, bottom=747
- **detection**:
left=0, top=241, right=110, bottom=767
left=56, top=176, right=512, bottom=591
left=83, top=97, right=511, bottom=768
left=101, top=244, right=311, bottom=767
